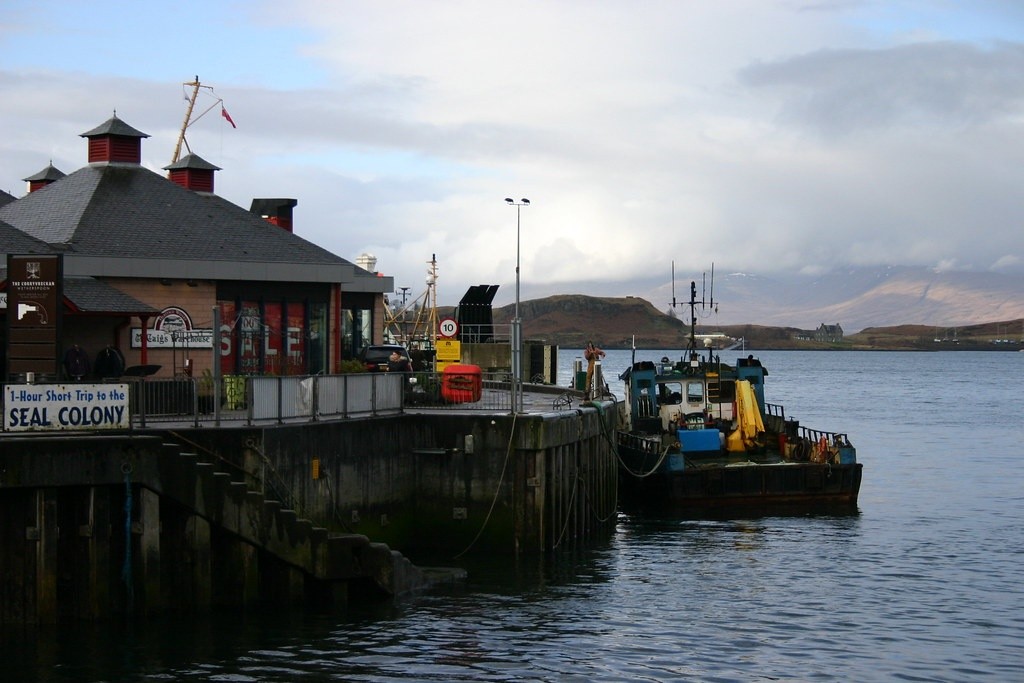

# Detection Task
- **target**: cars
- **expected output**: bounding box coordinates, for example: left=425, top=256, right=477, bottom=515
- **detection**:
left=360, top=346, right=415, bottom=376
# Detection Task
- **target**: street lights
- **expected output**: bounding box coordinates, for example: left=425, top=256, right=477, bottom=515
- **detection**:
left=504, top=195, right=530, bottom=413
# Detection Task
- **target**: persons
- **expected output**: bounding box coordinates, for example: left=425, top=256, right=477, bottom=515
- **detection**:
left=61, top=343, right=92, bottom=384
left=95, top=343, right=126, bottom=383
left=582, top=340, right=606, bottom=401
left=384, top=350, right=411, bottom=391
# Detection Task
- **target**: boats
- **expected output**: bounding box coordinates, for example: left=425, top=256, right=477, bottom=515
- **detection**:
left=617, top=260, right=863, bottom=509
